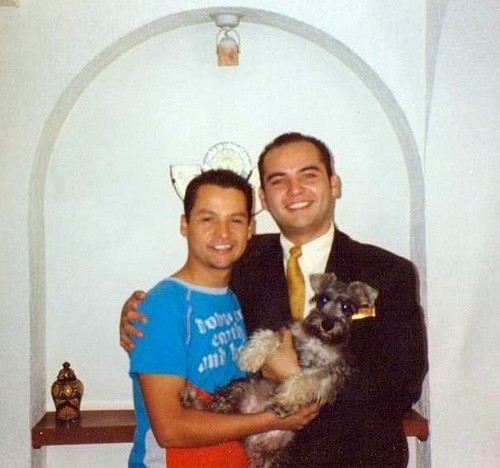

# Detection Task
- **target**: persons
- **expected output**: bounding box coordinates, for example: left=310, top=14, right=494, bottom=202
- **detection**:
left=128, top=170, right=322, bottom=468
left=119, top=133, right=429, bottom=468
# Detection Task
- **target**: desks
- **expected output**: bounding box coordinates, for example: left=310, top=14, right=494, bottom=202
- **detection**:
left=32, top=410, right=429, bottom=448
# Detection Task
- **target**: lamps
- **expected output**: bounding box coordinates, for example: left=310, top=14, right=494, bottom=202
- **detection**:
left=210, top=13, right=243, bottom=66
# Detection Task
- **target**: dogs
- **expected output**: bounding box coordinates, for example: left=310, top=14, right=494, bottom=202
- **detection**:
left=178, top=272, right=379, bottom=468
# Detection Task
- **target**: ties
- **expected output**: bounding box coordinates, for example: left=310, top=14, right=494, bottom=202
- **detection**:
left=285, top=247, right=306, bottom=319
